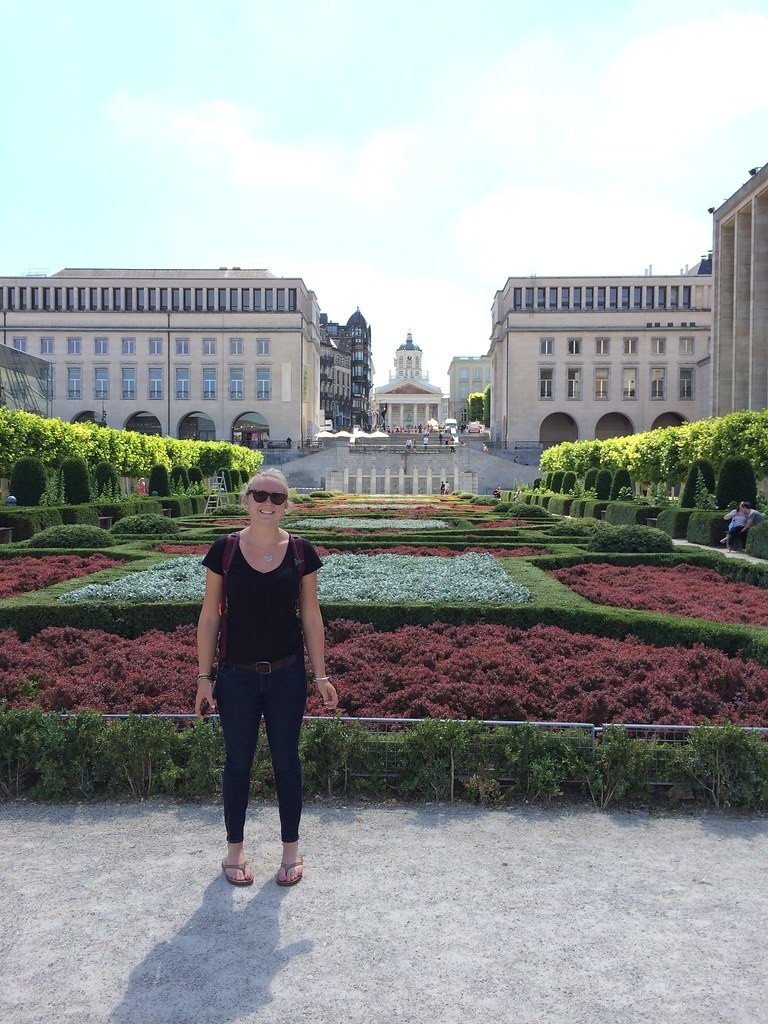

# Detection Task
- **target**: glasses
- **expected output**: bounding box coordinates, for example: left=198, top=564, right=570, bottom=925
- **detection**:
left=244, top=488, right=287, bottom=505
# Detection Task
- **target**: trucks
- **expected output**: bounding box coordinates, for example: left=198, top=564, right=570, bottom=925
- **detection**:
left=428, top=420, right=438, bottom=429
left=444, top=419, right=458, bottom=431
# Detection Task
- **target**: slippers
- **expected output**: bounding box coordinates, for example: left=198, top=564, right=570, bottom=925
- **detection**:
left=222, top=856, right=254, bottom=886
left=277, top=851, right=303, bottom=886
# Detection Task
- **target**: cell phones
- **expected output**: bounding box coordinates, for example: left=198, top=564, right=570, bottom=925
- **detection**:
left=201, top=679, right=216, bottom=716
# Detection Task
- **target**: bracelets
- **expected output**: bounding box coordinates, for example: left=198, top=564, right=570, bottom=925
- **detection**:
left=312, top=675, right=332, bottom=684
left=195, top=673, right=213, bottom=685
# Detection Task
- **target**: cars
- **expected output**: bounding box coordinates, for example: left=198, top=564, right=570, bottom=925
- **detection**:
left=468, top=422, right=480, bottom=433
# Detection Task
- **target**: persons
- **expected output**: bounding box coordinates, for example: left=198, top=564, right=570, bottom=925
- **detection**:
left=483, top=444, right=489, bottom=454
left=719, top=500, right=746, bottom=553
left=443, top=480, right=449, bottom=496
left=364, top=420, right=466, bottom=455
left=191, top=467, right=340, bottom=887
left=738, top=502, right=764, bottom=555
left=439, top=481, right=445, bottom=495
left=514, top=456, right=518, bottom=463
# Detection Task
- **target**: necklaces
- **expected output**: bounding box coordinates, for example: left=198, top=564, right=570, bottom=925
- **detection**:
left=248, top=527, right=282, bottom=563
